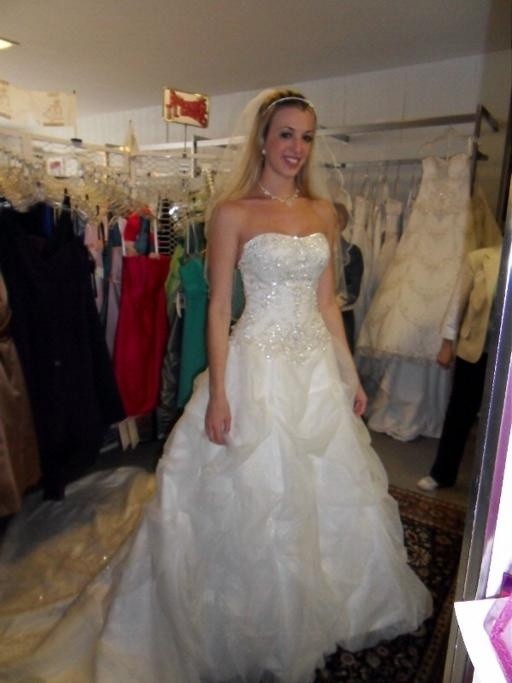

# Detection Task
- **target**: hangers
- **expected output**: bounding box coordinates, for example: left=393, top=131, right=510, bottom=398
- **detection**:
left=360, top=159, right=422, bottom=192
left=0, top=148, right=211, bottom=231
left=414, top=119, right=482, bottom=155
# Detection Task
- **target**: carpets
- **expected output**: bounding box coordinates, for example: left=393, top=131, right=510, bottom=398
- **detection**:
left=313, top=482, right=468, bottom=682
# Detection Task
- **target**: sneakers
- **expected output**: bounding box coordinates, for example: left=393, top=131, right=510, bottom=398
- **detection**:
left=416, top=472, right=438, bottom=490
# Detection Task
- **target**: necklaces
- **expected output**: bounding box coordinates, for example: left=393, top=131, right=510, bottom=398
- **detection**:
left=258, top=177, right=302, bottom=206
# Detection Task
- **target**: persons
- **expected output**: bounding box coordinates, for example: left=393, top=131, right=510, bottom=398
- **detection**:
left=330, top=197, right=362, bottom=356
left=417, top=237, right=508, bottom=490
left=0, top=86, right=436, bottom=681
